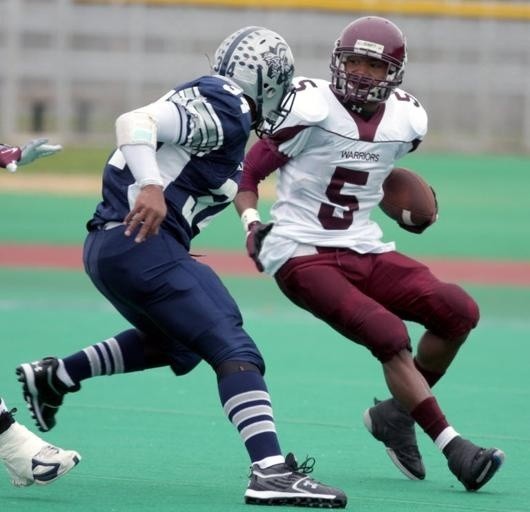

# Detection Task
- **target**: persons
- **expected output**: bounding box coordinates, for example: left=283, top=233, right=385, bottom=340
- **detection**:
left=0, top=138, right=81, bottom=486
left=233, top=16, right=503, bottom=492
left=17, top=25, right=347, bottom=508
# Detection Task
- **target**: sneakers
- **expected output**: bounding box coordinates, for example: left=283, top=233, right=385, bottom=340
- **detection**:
left=361, top=397, right=426, bottom=481
left=15, top=356, right=83, bottom=434
left=243, top=453, right=348, bottom=510
left=447, top=438, right=505, bottom=492
left=0, top=425, right=82, bottom=487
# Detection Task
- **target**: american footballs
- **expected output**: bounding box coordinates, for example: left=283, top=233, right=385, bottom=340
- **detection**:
left=378, top=168, right=436, bottom=226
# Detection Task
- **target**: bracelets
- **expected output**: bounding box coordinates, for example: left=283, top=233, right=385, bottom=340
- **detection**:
left=241, top=208, right=260, bottom=231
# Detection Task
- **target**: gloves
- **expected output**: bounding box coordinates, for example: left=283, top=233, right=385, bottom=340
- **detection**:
left=1, top=138, right=63, bottom=174
left=398, top=212, right=438, bottom=235
left=245, top=220, right=274, bottom=273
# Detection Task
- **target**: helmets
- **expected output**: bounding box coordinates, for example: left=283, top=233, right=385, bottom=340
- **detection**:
left=202, top=24, right=296, bottom=140
left=329, top=16, right=408, bottom=105
